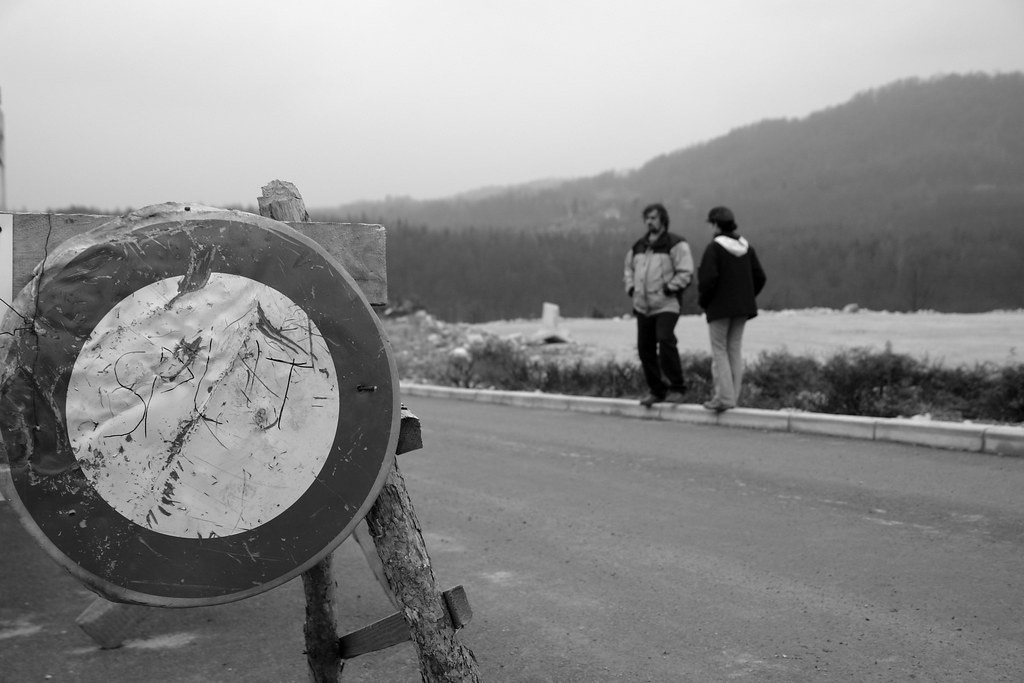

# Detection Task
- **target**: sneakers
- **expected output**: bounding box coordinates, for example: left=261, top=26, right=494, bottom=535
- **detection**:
left=641, top=392, right=688, bottom=405
left=704, top=399, right=734, bottom=410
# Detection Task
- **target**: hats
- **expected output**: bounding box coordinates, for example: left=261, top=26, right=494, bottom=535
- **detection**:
left=706, top=207, right=734, bottom=222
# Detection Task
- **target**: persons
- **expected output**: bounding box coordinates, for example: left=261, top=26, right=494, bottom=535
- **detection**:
left=623, top=203, right=694, bottom=407
left=696, top=206, right=768, bottom=410
